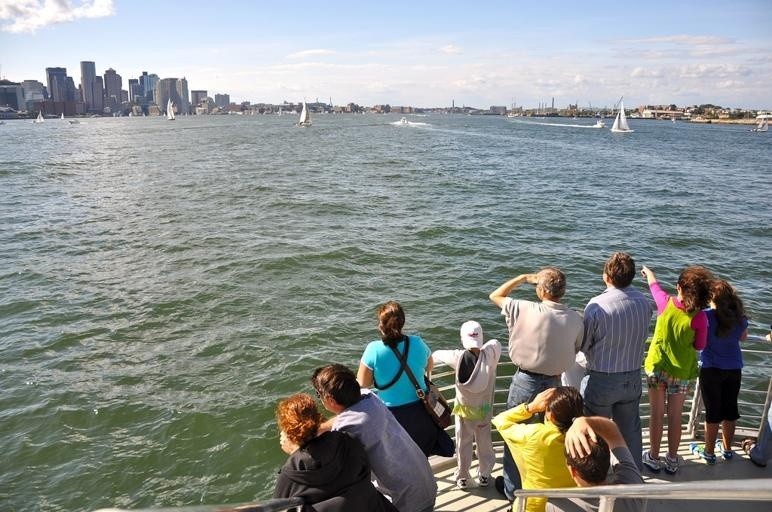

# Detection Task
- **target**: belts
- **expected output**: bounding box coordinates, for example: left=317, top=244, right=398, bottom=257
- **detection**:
left=517, top=367, right=561, bottom=378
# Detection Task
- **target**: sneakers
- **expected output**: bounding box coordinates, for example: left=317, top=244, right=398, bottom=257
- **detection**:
left=689, top=443, right=716, bottom=465
left=494, top=476, right=506, bottom=499
left=479, top=475, right=489, bottom=488
left=641, top=451, right=661, bottom=473
left=456, top=477, right=467, bottom=488
left=742, top=438, right=767, bottom=469
left=664, top=452, right=682, bottom=474
left=715, top=438, right=733, bottom=459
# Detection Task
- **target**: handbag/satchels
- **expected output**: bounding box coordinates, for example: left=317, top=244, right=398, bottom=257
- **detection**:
left=423, top=383, right=453, bottom=430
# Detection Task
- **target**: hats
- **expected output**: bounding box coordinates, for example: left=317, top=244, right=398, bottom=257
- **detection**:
left=459, top=320, right=484, bottom=351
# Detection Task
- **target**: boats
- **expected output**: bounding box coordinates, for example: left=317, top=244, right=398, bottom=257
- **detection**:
left=68, top=119, right=80, bottom=124
left=592, top=119, right=606, bottom=129
left=400, top=117, right=408, bottom=124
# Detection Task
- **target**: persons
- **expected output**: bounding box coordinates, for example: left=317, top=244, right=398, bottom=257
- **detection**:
left=640, top=264, right=713, bottom=474
left=277, top=394, right=398, bottom=512
left=696, top=279, right=748, bottom=463
left=312, top=364, right=437, bottom=511
left=356, top=300, right=433, bottom=458
left=431, top=320, right=502, bottom=490
left=749, top=326, right=772, bottom=468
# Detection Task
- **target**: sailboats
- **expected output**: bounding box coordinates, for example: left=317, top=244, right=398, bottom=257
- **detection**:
left=166, top=99, right=176, bottom=121
left=298, top=99, right=313, bottom=127
left=60, top=113, right=65, bottom=120
left=35, top=109, right=46, bottom=124
left=608, top=96, right=634, bottom=134
left=748, top=111, right=770, bottom=133
